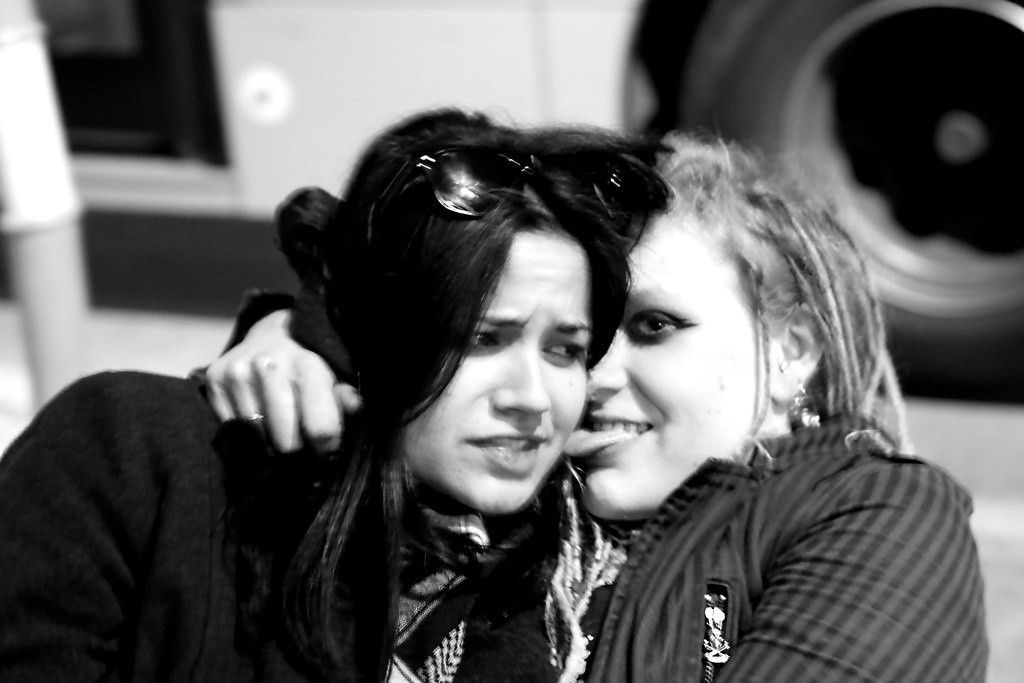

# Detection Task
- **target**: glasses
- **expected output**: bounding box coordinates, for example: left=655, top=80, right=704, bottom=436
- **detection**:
left=366, top=143, right=667, bottom=256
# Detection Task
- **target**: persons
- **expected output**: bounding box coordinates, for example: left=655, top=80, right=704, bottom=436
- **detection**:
left=0, top=110, right=676, bottom=683
left=0, top=1, right=99, bottom=410
left=204, top=132, right=989, bottom=683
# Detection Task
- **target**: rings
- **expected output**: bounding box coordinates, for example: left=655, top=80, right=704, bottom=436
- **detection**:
left=245, top=413, right=263, bottom=421
